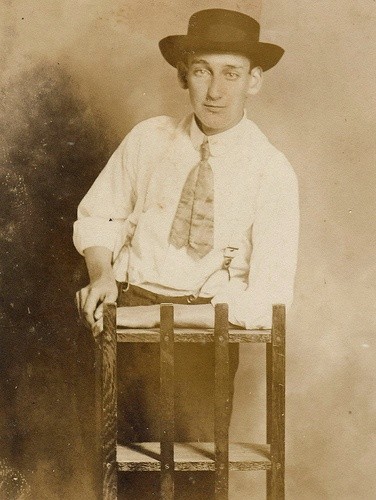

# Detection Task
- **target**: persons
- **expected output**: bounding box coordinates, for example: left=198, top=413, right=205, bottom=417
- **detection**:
left=69, top=5, right=302, bottom=500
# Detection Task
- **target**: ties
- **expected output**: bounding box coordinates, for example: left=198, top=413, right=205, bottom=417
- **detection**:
left=171, top=138, right=215, bottom=259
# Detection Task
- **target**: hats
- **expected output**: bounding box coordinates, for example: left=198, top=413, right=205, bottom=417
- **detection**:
left=155, top=7, right=283, bottom=74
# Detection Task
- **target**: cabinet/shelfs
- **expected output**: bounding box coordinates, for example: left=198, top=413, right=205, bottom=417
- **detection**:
left=90, top=301, right=287, bottom=500
